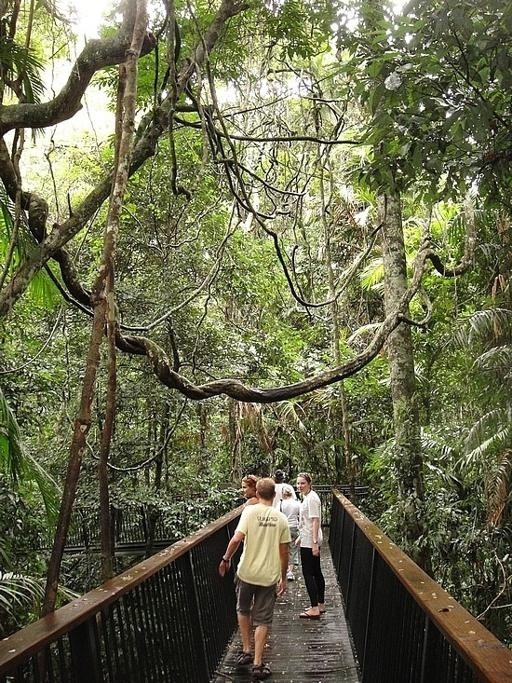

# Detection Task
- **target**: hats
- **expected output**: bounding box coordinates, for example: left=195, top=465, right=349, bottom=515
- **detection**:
left=282, top=486, right=292, bottom=494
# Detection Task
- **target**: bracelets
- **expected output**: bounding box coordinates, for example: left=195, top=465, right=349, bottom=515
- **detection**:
left=221, top=555, right=230, bottom=563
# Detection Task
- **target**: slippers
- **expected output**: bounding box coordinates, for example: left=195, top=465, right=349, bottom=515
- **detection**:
left=300, top=608, right=326, bottom=618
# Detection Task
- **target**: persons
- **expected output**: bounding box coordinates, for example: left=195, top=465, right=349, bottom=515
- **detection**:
left=218, top=477, right=293, bottom=680
left=241, top=467, right=327, bottom=620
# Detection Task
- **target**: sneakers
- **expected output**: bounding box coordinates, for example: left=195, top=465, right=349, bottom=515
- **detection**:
left=287, top=572, right=295, bottom=580
left=251, top=663, right=271, bottom=679
left=236, top=652, right=253, bottom=664
